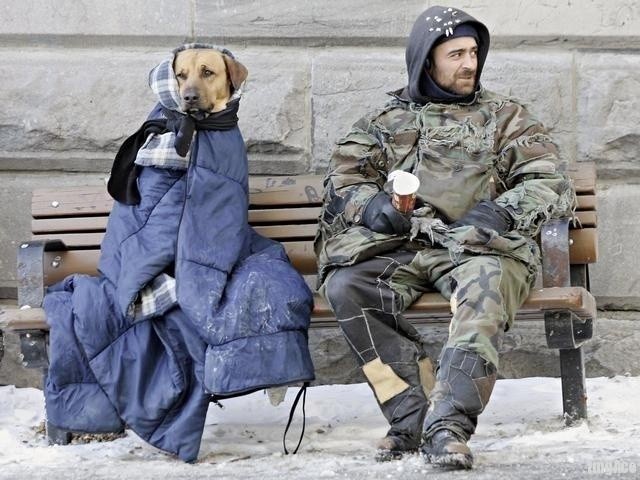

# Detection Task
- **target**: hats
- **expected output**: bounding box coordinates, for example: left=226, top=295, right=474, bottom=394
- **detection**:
left=427, top=21, right=483, bottom=53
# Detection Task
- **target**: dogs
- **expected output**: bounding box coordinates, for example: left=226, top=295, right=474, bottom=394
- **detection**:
left=172, top=46, right=249, bottom=120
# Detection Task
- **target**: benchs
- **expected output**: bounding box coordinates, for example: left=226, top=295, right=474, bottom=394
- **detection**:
left=0, top=162, right=598, bottom=445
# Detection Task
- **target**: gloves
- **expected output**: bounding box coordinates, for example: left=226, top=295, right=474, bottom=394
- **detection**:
left=453, top=198, right=514, bottom=240
left=365, top=190, right=412, bottom=236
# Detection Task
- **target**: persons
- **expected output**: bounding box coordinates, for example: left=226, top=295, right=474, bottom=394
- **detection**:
left=311, top=3, right=575, bottom=474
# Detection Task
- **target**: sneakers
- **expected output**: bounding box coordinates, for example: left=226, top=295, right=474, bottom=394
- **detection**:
left=420, top=427, right=473, bottom=470
left=375, top=429, right=421, bottom=462
